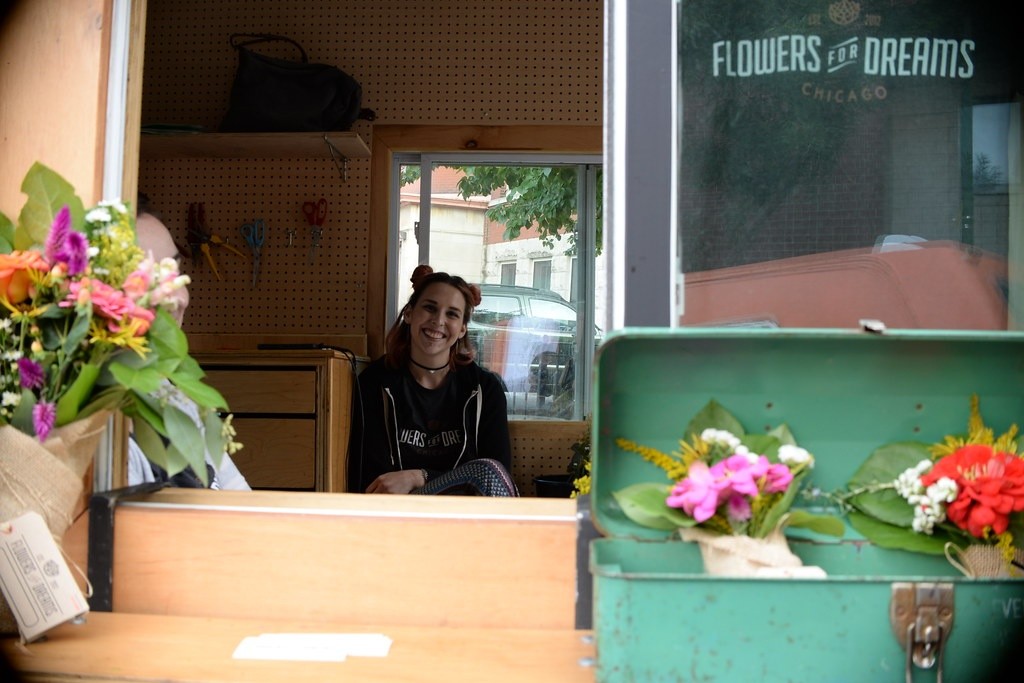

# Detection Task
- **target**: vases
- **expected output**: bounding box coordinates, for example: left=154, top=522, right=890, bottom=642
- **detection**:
left=536, top=473, right=574, bottom=498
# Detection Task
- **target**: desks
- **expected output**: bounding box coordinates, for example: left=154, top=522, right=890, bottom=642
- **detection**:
left=1, top=610, right=593, bottom=683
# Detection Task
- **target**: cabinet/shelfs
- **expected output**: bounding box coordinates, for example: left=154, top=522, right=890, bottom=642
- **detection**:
left=188, top=352, right=370, bottom=492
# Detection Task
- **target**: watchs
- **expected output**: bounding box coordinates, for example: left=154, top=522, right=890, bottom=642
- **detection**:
left=421, top=469, right=428, bottom=482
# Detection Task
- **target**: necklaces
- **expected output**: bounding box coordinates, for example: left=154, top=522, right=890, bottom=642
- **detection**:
left=409, top=356, right=450, bottom=374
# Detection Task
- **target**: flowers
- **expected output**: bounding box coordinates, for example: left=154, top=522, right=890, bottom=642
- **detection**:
left=0, top=161, right=243, bottom=489
left=840, top=389, right=1024, bottom=578
left=567, top=417, right=590, bottom=500
left=609, top=401, right=847, bottom=538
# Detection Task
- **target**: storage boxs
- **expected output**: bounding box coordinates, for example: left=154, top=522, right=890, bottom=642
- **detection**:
left=591, top=328, right=1024, bottom=683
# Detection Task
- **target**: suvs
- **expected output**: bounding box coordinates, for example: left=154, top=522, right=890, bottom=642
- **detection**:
left=448, top=283, right=602, bottom=395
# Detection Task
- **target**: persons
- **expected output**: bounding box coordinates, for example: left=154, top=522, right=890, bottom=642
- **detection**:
left=127, top=208, right=256, bottom=490
left=344, top=264, right=523, bottom=498
